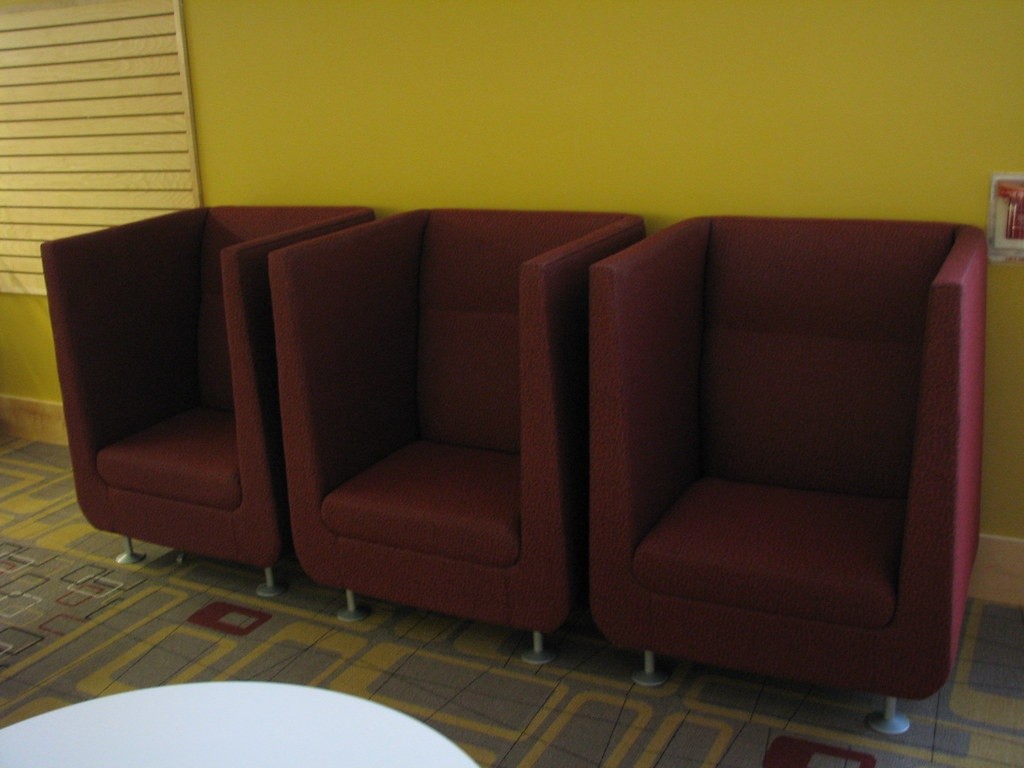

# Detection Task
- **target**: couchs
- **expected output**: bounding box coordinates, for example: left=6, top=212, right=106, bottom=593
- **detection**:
left=586, top=215, right=988, bottom=734
left=267, top=207, right=645, bottom=666
left=40, top=205, right=374, bottom=598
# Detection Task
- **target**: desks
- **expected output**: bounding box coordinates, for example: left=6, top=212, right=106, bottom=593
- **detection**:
left=0, top=680, right=479, bottom=768
